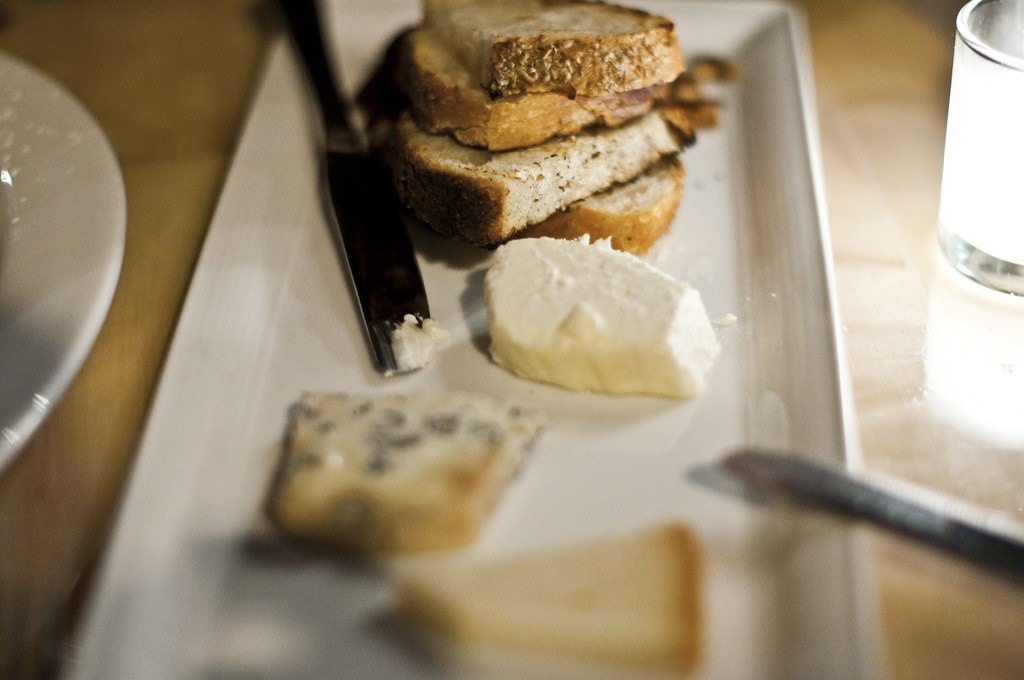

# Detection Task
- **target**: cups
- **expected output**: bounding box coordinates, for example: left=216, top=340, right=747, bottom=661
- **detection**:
left=934, top=0, right=1024, bottom=293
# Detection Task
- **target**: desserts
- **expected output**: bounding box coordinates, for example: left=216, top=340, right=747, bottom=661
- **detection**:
left=476, top=232, right=721, bottom=400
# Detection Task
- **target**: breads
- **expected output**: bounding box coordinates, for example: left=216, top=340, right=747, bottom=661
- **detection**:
left=397, top=517, right=712, bottom=680
left=385, top=0, right=699, bottom=258
left=264, top=386, right=545, bottom=549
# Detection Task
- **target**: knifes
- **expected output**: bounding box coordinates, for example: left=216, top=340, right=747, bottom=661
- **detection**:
left=275, top=1, right=434, bottom=377
left=694, top=443, right=1024, bottom=585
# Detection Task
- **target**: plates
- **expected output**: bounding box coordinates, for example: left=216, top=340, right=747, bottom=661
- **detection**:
left=0, top=53, right=127, bottom=471
left=62, top=1, right=887, bottom=680
